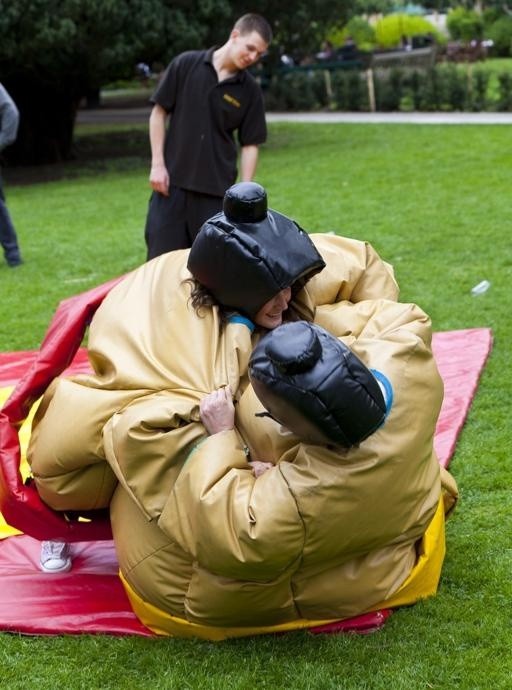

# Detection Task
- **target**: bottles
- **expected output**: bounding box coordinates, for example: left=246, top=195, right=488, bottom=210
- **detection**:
left=471, top=280, right=489, bottom=297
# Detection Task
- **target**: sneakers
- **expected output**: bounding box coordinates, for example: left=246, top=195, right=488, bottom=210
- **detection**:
left=7, top=256, right=23, bottom=268
left=40, top=540, right=72, bottom=574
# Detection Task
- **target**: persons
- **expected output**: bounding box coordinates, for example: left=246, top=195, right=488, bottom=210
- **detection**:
left=249, top=32, right=495, bottom=111
left=108, top=296, right=458, bottom=643
left=145, top=12, right=268, bottom=262
left=0, top=81, right=22, bottom=268
left=0, top=179, right=401, bottom=574
left=134, top=62, right=150, bottom=87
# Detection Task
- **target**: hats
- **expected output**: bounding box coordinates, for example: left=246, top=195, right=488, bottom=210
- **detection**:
left=187, top=181, right=326, bottom=324
left=247, top=318, right=387, bottom=452
